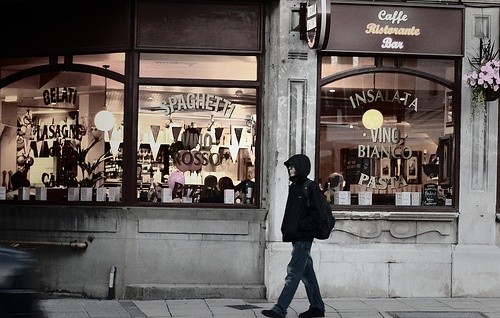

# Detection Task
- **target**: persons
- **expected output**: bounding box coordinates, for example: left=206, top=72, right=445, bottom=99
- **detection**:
left=260, top=154, right=325, bottom=318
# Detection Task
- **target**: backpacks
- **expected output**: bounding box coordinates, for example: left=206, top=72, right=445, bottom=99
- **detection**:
left=303, top=180, right=335, bottom=239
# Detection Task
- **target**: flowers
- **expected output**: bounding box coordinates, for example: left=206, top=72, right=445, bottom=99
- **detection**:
left=467, top=40, right=500, bottom=92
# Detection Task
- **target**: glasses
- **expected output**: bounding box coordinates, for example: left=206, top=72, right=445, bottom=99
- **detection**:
left=289, top=164, right=294, bottom=169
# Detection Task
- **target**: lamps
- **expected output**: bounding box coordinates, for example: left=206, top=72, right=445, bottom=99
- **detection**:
left=95, top=63, right=116, bottom=131
left=362, top=73, right=384, bottom=130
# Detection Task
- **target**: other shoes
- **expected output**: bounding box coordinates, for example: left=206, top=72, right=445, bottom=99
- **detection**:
left=262, top=310, right=285, bottom=318
left=299, top=307, right=325, bottom=318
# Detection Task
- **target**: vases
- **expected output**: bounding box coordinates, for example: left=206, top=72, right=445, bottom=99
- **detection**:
left=482, top=87, right=500, bottom=102
